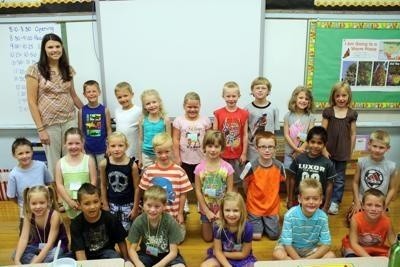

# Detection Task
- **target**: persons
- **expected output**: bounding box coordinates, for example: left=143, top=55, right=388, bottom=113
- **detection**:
left=7, top=126, right=400, bottom=267
left=114, top=82, right=141, bottom=173
left=212, top=81, right=249, bottom=193
left=283, top=86, right=315, bottom=209
left=138, top=88, right=172, bottom=169
left=173, top=92, right=212, bottom=213
left=242, top=77, right=281, bottom=165
left=25, top=34, right=83, bottom=213
left=78, top=80, right=113, bottom=175
left=322, top=82, right=358, bottom=214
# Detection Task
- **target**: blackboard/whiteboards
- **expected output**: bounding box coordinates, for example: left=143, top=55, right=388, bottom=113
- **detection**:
left=0, top=12, right=400, bottom=130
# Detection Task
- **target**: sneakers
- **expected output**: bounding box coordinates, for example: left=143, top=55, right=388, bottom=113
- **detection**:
left=327, top=200, right=339, bottom=214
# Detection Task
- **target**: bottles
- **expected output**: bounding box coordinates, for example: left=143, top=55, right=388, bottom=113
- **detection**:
left=389, top=233, right=400, bottom=266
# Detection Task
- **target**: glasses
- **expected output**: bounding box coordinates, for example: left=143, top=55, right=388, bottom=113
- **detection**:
left=257, top=144, right=276, bottom=150
